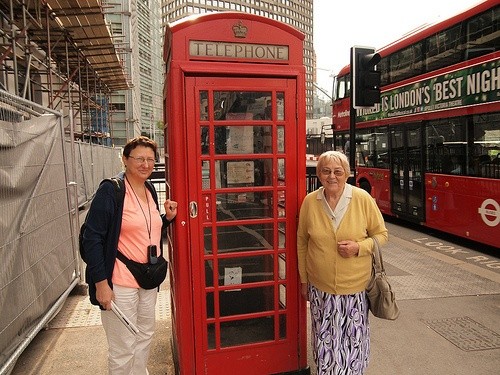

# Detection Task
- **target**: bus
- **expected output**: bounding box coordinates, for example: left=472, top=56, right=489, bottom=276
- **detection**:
left=306, top=0, right=500, bottom=249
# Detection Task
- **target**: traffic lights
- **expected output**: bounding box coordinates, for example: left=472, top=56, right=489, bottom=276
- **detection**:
left=353, top=46, right=382, bottom=109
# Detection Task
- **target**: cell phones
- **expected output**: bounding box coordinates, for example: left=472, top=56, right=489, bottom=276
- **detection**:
left=148, top=244, right=158, bottom=265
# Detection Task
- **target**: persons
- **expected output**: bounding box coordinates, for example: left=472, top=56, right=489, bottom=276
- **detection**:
left=85, top=136, right=178, bottom=375
left=297, top=151, right=388, bottom=375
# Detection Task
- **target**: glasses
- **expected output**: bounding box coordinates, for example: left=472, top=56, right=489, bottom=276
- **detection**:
left=127, top=155, right=155, bottom=163
left=320, top=169, right=345, bottom=176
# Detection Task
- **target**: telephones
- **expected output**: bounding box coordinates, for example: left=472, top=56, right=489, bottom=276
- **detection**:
left=202, top=156, right=264, bottom=212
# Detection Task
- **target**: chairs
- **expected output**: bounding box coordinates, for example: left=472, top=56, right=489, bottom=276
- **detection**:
left=479, top=155, right=500, bottom=163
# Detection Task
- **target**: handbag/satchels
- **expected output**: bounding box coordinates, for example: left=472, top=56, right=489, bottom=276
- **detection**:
left=366, top=236, right=400, bottom=321
left=116, top=250, right=168, bottom=290
